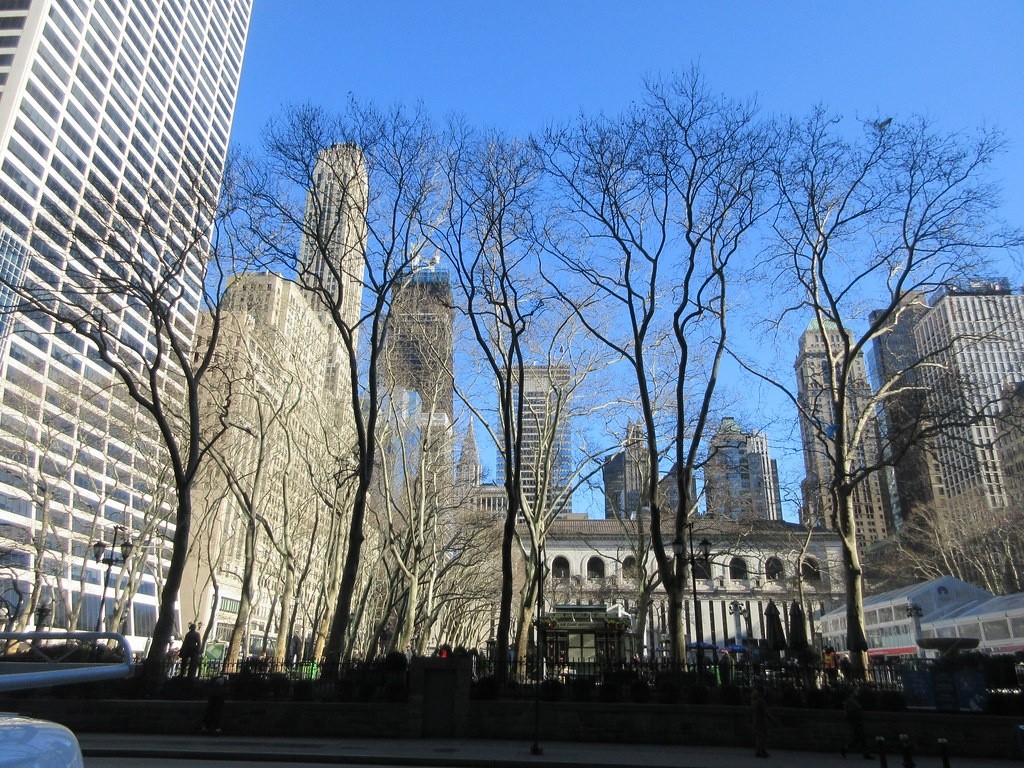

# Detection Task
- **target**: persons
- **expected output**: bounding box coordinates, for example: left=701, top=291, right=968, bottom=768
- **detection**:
left=823, top=645, right=839, bottom=686
left=839, top=687, right=877, bottom=761
left=177, top=623, right=201, bottom=676
left=258, top=650, right=272, bottom=679
left=200, top=677, right=223, bottom=736
left=750, top=684, right=778, bottom=758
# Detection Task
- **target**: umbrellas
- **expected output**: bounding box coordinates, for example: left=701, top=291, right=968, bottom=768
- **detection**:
left=687, top=641, right=749, bottom=654
left=763, top=597, right=812, bottom=688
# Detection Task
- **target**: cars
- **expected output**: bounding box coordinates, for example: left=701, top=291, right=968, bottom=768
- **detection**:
left=0, top=712, right=86, bottom=768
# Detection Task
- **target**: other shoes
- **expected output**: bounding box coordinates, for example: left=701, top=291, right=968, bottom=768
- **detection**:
left=863, top=754, right=875, bottom=758
left=841, top=744, right=846, bottom=758
left=756, top=751, right=768, bottom=757
left=763, top=751, right=769, bottom=756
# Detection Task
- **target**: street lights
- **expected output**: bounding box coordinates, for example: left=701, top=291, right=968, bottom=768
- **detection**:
left=89, top=523, right=134, bottom=659
left=28, top=595, right=54, bottom=650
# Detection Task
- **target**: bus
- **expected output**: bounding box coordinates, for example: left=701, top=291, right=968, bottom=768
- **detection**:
left=223, top=631, right=278, bottom=663
